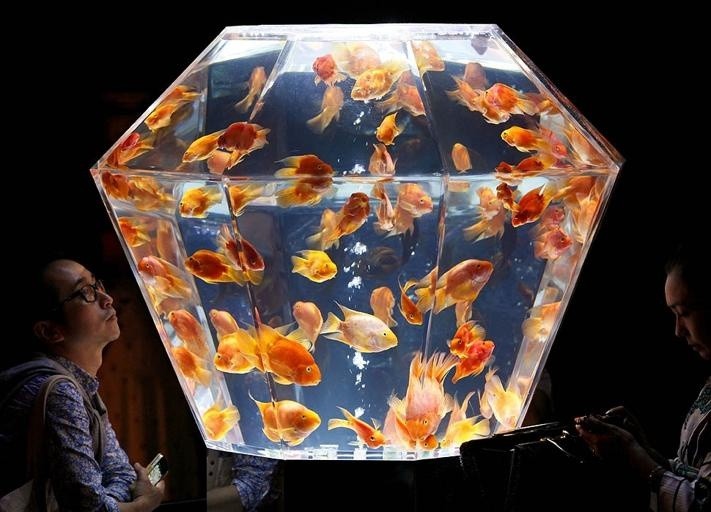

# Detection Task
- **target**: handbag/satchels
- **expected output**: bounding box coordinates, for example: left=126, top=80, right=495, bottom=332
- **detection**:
left=1, top=374, right=105, bottom=511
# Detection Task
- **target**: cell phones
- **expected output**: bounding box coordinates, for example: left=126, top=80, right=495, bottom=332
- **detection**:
left=129, top=453, right=169, bottom=502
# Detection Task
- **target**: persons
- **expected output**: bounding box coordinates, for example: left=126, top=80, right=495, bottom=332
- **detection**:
left=572, top=251, right=711, bottom=511
left=522, top=370, right=551, bottom=428
left=206, top=449, right=285, bottom=510
left=1, top=254, right=170, bottom=512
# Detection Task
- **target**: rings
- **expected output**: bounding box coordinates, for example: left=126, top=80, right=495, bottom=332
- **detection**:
left=590, top=448, right=594, bottom=456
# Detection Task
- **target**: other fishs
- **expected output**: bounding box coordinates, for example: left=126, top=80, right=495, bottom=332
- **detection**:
left=98, top=39, right=611, bottom=452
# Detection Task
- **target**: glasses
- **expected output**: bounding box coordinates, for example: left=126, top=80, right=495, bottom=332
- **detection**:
left=54, top=279, right=106, bottom=311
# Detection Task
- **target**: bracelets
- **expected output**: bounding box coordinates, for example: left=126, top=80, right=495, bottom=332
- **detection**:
left=648, top=465, right=663, bottom=480
